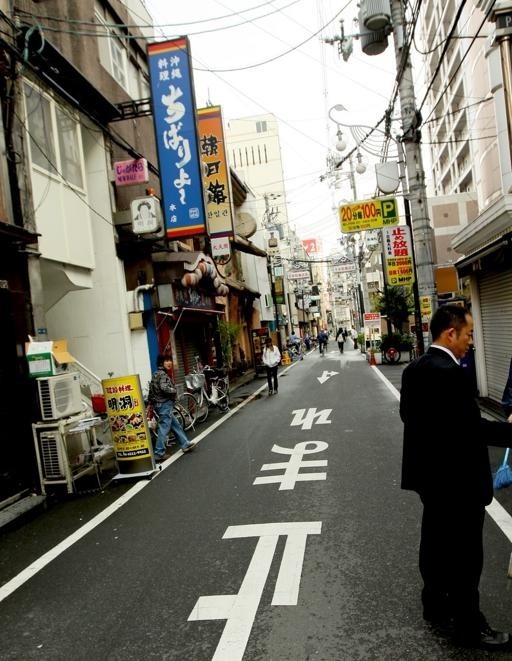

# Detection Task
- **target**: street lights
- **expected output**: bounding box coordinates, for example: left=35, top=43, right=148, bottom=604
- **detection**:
left=328, top=103, right=425, bottom=355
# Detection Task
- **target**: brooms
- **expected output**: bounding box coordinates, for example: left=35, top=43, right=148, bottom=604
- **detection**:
left=493, top=448, right=512, bottom=491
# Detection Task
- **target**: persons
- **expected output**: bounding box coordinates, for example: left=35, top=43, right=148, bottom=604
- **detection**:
left=351, top=325, right=359, bottom=349
left=147, top=353, right=196, bottom=462
left=287, top=327, right=348, bottom=361
left=134, top=202, right=156, bottom=220
left=261, top=337, right=281, bottom=394
left=398, top=305, right=511, bottom=651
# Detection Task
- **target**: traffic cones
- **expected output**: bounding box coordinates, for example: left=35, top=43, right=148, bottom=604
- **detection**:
left=369, top=352, right=377, bottom=365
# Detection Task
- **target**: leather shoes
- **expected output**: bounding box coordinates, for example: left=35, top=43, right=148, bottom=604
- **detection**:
left=466, top=626, right=511, bottom=650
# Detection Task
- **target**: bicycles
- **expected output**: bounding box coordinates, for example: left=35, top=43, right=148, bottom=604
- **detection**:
left=289, top=335, right=327, bottom=361
left=146, top=355, right=229, bottom=447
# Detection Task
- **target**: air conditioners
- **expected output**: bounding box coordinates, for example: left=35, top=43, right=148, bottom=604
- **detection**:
left=37, top=372, right=94, bottom=477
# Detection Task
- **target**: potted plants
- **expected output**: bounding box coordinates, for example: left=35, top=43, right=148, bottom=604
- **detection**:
left=217, top=321, right=242, bottom=383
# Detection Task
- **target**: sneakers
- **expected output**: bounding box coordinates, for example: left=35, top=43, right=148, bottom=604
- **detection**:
left=182, top=442, right=196, bottom=452
left=155, top=454, right=170, bottom=460
left=269, top=389, right=278, bottom=395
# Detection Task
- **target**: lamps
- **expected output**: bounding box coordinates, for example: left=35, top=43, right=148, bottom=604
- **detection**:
left=328, top=104, right=407, bottom=174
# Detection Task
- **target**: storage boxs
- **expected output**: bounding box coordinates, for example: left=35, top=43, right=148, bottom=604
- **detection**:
left=17, top=336, right=77, bottom=378
